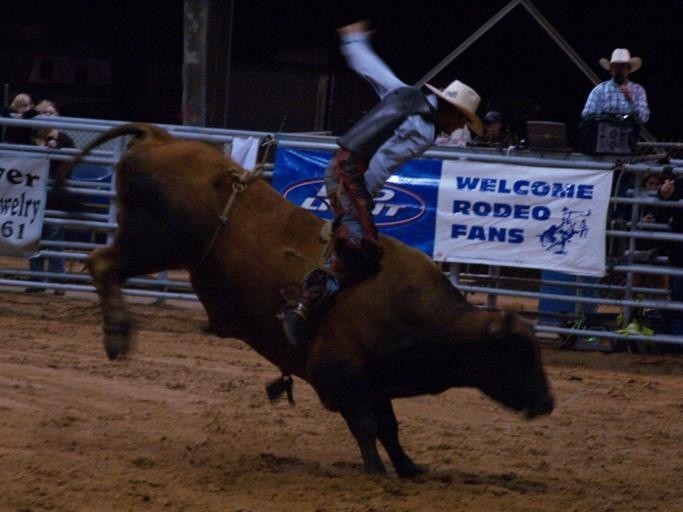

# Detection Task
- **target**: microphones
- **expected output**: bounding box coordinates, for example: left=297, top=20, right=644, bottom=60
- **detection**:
left=614, top=72, right=632, bottom=102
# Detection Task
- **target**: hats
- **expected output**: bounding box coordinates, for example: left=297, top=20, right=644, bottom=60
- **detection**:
left=599, top=48, right=643, bottom=74
left=424, top=80, right=487, bottom=139
left=482, top=111, right=503, bottom=126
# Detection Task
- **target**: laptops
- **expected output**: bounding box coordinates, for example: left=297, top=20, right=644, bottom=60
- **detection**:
left=527, top=120, right=565, bottom=150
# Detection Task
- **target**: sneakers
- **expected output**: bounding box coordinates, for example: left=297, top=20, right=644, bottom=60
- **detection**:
left=283, top=304, right=316, bottom=348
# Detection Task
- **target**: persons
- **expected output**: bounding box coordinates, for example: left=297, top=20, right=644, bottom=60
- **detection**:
left=1, top=95, right=78, bottom=296
left=427, top=47, right=683, bottom=322
left=277, top=21, right=483, bottom=346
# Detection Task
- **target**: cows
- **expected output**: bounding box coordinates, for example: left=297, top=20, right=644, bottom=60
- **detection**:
left=50, top=122, right=556, bottom=486
